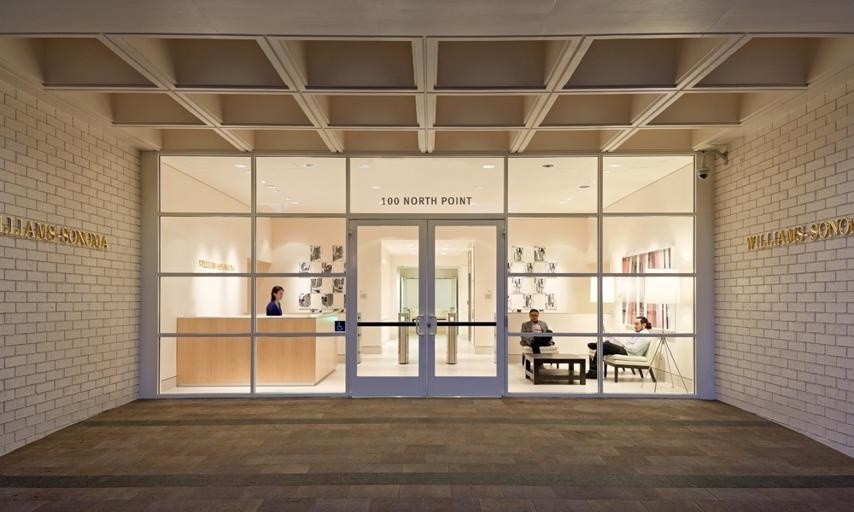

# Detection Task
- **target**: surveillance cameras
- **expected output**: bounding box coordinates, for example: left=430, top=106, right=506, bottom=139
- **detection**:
left=698, top=167, right=711, bottom=179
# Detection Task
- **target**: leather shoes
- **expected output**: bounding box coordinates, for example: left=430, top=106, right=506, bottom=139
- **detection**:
left=586, top=374, right=591, bottom=379
left=588, top=343, right=597, bottom=349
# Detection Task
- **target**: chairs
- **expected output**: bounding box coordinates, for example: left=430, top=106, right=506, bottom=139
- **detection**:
left=521, top=328, right=659, bottom=382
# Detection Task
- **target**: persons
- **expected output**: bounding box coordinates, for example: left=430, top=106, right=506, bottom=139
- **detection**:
left=265, top=285, right=284, bottom=316
left=585, top=316, right=651, bottom=379
left=521, top=308, right=552, bottom=368
